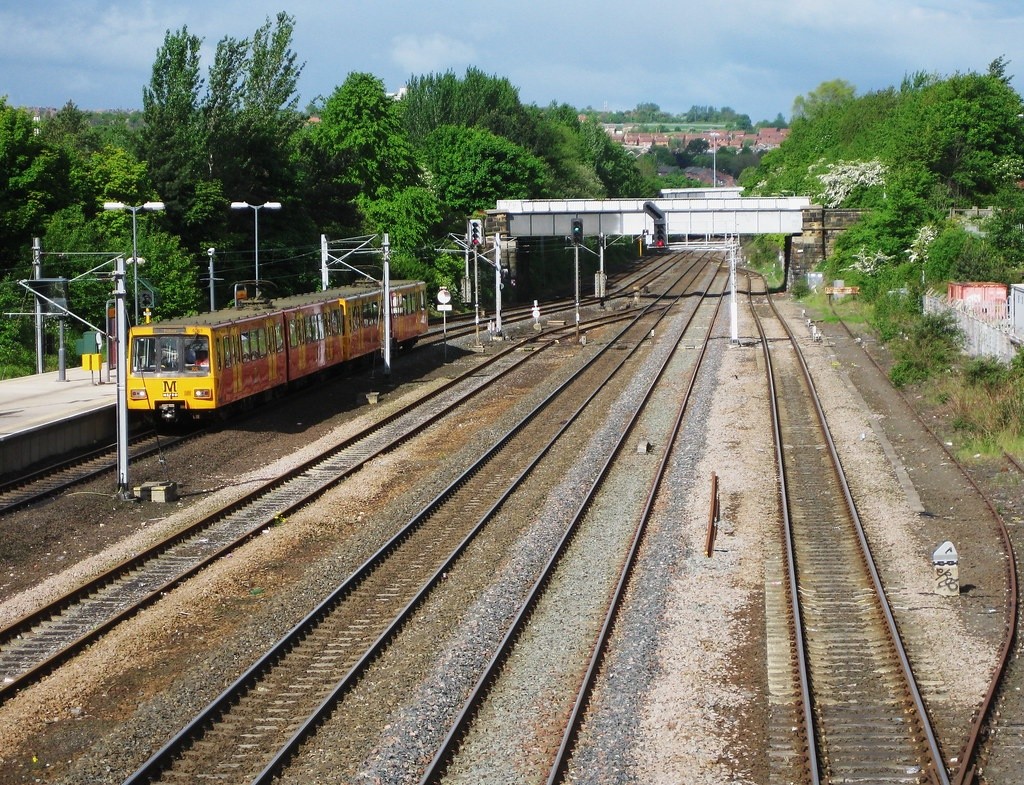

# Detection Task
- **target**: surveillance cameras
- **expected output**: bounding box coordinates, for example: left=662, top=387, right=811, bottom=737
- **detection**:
left=207, top=248, right=215, bottom=255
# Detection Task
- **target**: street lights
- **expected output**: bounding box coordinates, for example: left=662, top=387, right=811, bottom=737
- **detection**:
left=709, top=132, right=721, bottom=188
left=230, top=199, right=281, bottom=300
left=105, top=199, right=165, bottom=327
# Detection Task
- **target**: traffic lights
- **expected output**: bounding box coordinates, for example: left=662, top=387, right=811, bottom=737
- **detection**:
left=469, top=219, right=484, bottom=247
left=654, top=223, right=667, bottom=247
left=571, top=217, right=584, bottom=245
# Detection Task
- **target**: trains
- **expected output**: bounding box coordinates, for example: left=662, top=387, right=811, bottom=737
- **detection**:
left=127, top=280, right=430, bottom=434
